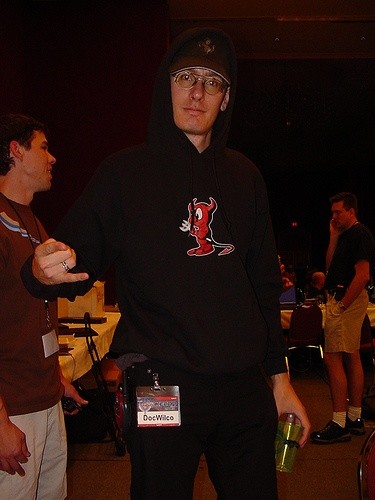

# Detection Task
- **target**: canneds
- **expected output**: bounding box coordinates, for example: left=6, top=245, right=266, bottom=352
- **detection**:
left=275, top=413, right=303, bottom=473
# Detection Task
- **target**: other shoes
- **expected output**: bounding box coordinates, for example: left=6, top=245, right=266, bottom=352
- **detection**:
left=346, top=415, right=366, bottom=436
left=310, top=419, right=351, bottom=444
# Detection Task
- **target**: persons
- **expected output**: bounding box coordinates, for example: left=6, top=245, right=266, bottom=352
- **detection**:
left=306, top=271, right=325, bottom=302
left=0, top=112, right=88, bottom=500
left=310, top=193, right=373, bottom=443
left=31, top=27, right=310, bottom=500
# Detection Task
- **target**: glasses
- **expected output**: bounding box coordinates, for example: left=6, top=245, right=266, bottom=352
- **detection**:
left=172, top=69, right=226, bottom=95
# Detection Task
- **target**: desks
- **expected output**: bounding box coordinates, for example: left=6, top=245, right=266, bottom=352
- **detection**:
left=55, top=312, right=121, bottom=384
left=277, top=300, right=375, bottom=330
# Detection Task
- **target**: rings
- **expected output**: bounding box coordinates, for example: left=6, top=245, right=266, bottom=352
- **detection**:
left=62, top=261, right=70, bottom=272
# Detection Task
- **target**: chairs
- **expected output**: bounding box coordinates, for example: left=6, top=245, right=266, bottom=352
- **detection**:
left=281, top=302, right=375, bottom=384
left=78, top=309, right=126, bottom=457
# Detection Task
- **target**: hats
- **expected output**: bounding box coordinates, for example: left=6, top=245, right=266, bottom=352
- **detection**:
left=167, top=26, right=232, bottom=86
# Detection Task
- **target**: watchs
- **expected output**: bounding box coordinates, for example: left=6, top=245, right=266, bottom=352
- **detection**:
left=338, top=301, right=347, bottom=309
